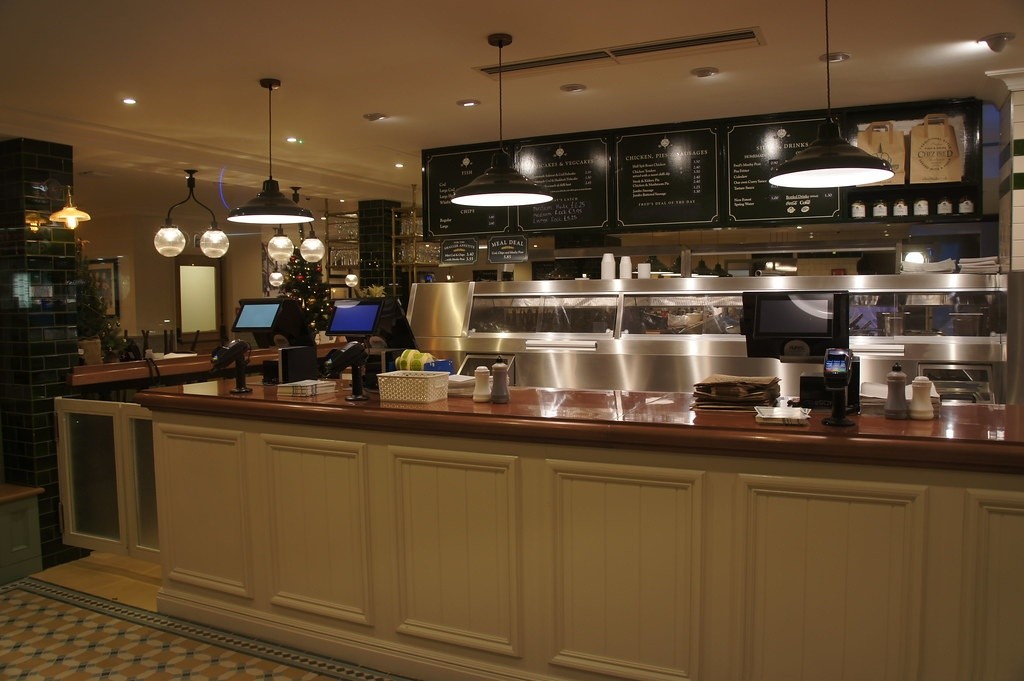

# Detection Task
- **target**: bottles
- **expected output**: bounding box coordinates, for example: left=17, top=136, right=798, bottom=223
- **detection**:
left=883, top=362, right=908, bottom=420
left=490, top=356, right=509, bottom=404
left=472, top=366, right=492, bottom=402
left=909, top=376, right=934, bottom=420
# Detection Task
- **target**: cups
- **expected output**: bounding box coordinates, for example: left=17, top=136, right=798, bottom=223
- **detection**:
left=403, top=242, right=441, bottom=263
left=600, top=253, right=616, bottom=280
left=619, top=256, right=632, bottom=279
left=331, top=249, right=359, bottom=265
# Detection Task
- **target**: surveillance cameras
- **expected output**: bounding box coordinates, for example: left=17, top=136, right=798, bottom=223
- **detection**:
left=986, top=36, right=1007, bottom=53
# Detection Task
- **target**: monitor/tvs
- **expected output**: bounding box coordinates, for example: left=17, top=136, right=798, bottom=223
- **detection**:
left=753, top=292, right=834, bottom=342
left=231, top=302, right=283, bottom=332
left=325, top=299, right=385, bottom=338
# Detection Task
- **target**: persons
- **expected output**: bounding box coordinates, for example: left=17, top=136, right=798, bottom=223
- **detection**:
left=853, top=256, right=896, bottom=329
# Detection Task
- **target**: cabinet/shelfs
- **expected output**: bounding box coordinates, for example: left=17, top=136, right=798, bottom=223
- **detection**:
left=324, top=184, right=441, bottom=314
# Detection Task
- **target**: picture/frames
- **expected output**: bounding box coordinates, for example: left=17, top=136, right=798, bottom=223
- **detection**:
left=88, top=258, right=120, bottom=318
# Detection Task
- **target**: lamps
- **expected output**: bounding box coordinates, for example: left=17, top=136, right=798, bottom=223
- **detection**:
left=713, top=229, right=733, bottom=277
left=269, top=186, right=326, bottom=263
left=25, top=212, right=48, bottom=233
left=767, top=0, right=895, bottom=188
left=345, top=269, right=358, bottom=287
left=269, top=261, right=284, bottom=287
left=153, top=169, right=229, bottom=259
left=691, top=229, right=720, bottom=277
left=226, top=79, right=314, bottom=225
left=49, top=183, right=91, bottom=230
left=660, top=232, right=699, bottom=276
left=631, top=232, right=674, bottom=274
left=450, top=33, right=553, bottom=207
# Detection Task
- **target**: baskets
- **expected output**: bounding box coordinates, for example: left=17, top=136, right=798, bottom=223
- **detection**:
left=376, top=370, right=451, bottom=404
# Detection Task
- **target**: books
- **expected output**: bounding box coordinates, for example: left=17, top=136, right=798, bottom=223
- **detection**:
left=902, top=258, right=956, bottom=275
left=446, top=373, right=495, bottom=397
left=278, top=378, right=338, bottom=398
left=958, top=256, right=1001, bottom=274
left=753, top=405, right=810, bottom=424
left=690, top=376, right=780, bottom=415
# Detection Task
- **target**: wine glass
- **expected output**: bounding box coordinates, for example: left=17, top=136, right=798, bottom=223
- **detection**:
left=332, top=219, right=360, bottom=240
